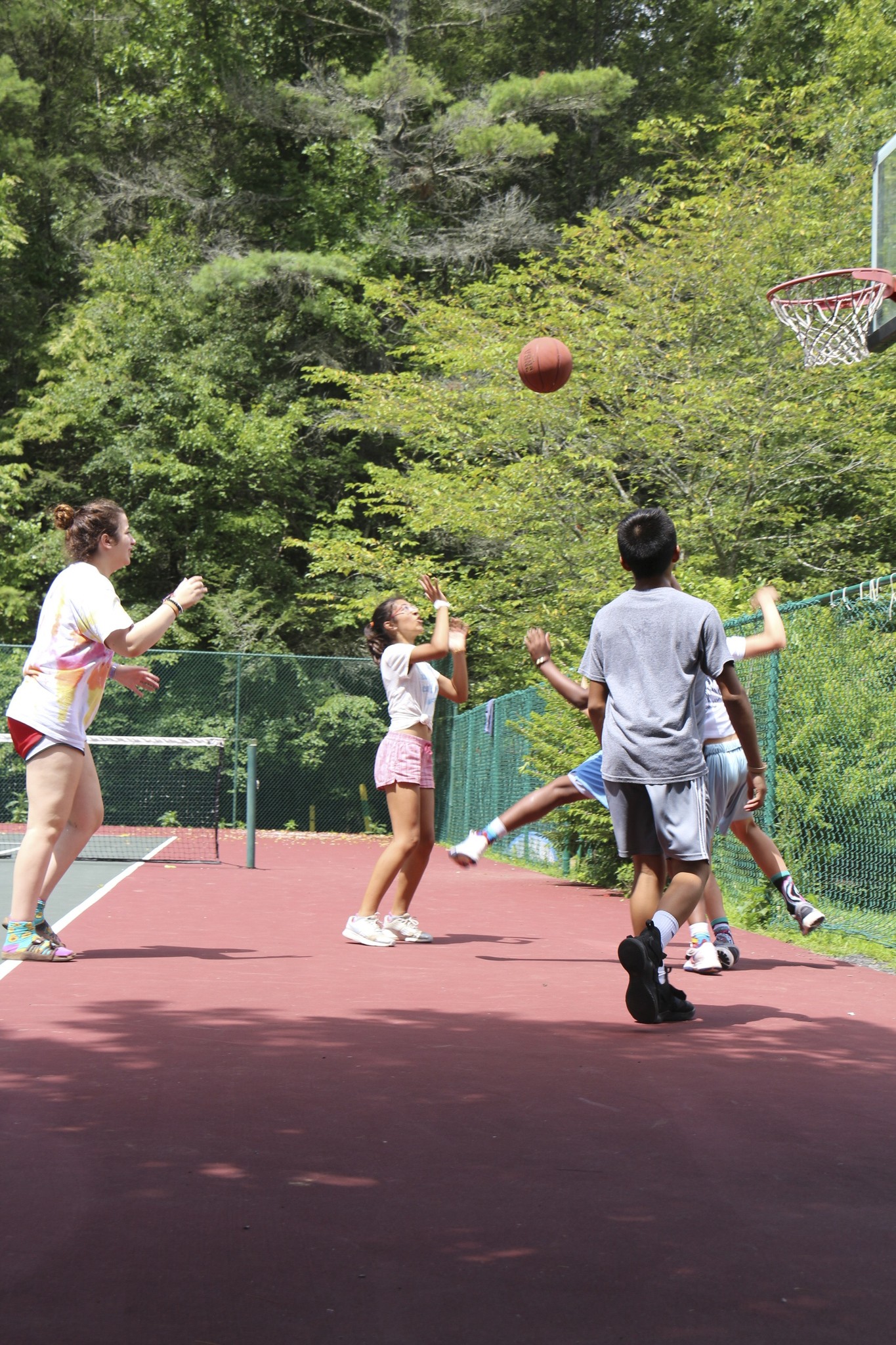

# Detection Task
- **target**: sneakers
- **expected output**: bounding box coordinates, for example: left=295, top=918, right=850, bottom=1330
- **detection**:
left=342, top=912, right=433, bottom=946
left=618, top=920, right=668, bottom=1023
left=448, top=829, right=488, bottom=867
left=683, top=942, right=722, bottom=973
left=792, top=901, right=825, bottom=936
left=713, top=939, right=740, bottom=970
left=655, top=966, right=695, bottom=1023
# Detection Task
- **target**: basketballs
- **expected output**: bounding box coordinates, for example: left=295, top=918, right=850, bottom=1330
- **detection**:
left=518, top=337, right=573, bottom=394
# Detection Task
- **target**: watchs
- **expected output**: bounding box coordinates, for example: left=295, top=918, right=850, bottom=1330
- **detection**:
left=433, top=600, right=450, bottom=610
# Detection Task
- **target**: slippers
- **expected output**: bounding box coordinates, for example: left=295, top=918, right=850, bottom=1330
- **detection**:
left=1, top=937, right=77, bottom=962
left=1, top=917, right=66, bottom=948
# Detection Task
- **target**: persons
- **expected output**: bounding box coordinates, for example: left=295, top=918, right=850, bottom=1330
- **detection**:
left=342, top=574, right=469, bottom=947
left=578, top=508, right=767, bottom=1024
left=2, top=500, right=208, bottom=962
left=447, top=586, right=826, bottom=975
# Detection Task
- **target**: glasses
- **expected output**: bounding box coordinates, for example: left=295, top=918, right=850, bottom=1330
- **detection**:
left=387, top=604, right=419, bottom=621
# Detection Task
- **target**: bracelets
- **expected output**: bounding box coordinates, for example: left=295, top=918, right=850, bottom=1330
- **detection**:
left=163, top=594, right=183, bottom=618
left=536, top=655, right=550, bottom=665
left=110, top=663, right=116, bottom=678
left=451, top=648, right=466, bottom=653
left=747, top=763, right=766, bottom=773
